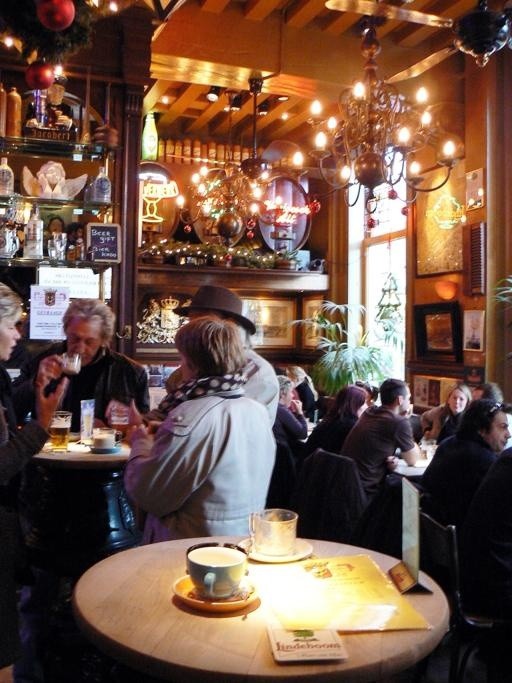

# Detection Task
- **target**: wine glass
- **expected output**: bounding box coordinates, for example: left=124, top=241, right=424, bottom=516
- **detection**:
left=51, top=231, right=68, bottom=260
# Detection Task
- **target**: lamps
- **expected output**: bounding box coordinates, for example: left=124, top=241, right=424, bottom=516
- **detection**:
left=229, top=94, right=243, bottom=111
left=175, top=75, right=272, bottom=241
left=223, top=75, right=320, bottom=226
left=206, top=86, right=221, bottom=102
left=258, top=100, right=268, bottom=117
left=292, top=23, right=464, bottom=215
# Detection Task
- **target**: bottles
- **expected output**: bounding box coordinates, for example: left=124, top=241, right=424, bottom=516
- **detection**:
left=27, top=203, right=44, bottom=257
left=7, top=88, right=22, bottom=138
left=0, top=157, right=14, bottom=195
left=142, top=111, right=158, bottom=161
left=93, top=166, right=112, bottom=203
left=0, top=81, right=6, bottom=137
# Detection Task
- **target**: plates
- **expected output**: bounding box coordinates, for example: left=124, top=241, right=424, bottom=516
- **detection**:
left=39, top=441, right=78, bottom=454
left=238, top=538, right=313, bottom=564
left=173, top=576, right=260, bottom=612
left=139, top=255, right=165, bottom=264
left=90, top=447, right=124, bottom=455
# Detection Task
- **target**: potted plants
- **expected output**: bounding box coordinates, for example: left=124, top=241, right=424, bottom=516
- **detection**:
left=139, top=234, right=294, bottom=269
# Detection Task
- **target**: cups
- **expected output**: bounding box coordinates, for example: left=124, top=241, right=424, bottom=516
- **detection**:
left=246, top=509, right=298, bottom=557
left=421, top=438, right=436, bottom=451
left=46, top=410, right=72, bottom=450
left=81, top=399, right=94, bottom=442
left=47, top=239, right=55, bottom=259
left=185, top=542, right=249, bottom=599
left=110, top=396, right=128, bottom=425
left=92, top=427, right=123, bottom=450
left=62, top=353, right=82, bottom=374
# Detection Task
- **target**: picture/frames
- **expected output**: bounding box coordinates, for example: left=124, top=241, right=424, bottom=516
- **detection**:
left=87, top=223, right=121, bottom=263
left=412, top=156, right=466, bottom=279
left=132, top=283, right=199, bottom=356
left=239, top=297, right=298, bottom=349
left=301, top=296, right=324, bottom=348
left=413, top=301, right=464, bottom=365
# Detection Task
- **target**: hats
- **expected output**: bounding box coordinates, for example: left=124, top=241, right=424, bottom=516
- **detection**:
left=173, top=285, right=256, bottom=335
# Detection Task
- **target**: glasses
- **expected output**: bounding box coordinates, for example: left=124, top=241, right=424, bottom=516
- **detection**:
left=491, top=401, right=502, bottom=413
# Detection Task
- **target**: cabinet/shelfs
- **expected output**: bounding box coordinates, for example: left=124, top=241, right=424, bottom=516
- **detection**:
left=0, top=0, right=145, bottom=366
left=137, top=262, right=329, bottom=292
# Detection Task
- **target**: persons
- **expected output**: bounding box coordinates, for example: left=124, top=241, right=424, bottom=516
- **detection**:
left=420, top=385, right=474, bottom=443
left=466, top=446, right=512, bottom=683
left=270, top=374, right=307, bottom=507
left=124, top=315, right=277, bottom=544
left=0, top=282, right=64, bottom=683
left=282, top=365, right=318, bottom=413
left=339, top=378, right=420, bottom=552
left=162, top=285, right=280, bottom=431
left=471, top=383, right=504, bottom=405
left=11, top=297, right=149, bottom=424
left=303, top=384, right=369, bottom=461
left=422, top=398, right=512, bottom=557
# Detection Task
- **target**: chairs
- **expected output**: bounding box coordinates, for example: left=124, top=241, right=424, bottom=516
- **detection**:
left=418, top=507, right=512, bottom=683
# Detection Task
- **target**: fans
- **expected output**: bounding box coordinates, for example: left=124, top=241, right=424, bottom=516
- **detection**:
left=323, top=0, right=511, bottom=86
